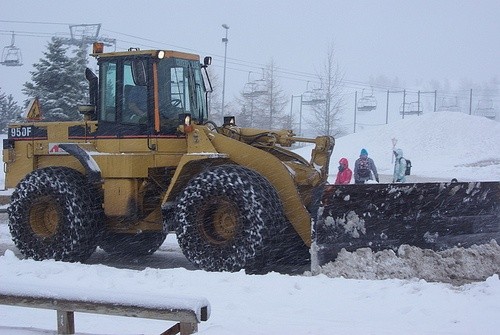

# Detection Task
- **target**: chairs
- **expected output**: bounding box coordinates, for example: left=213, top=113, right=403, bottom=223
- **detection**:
left=125, top=85, right=134, bottom=108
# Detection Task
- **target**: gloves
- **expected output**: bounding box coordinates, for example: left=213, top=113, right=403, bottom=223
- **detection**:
left=377, top=178, right=379, bottom=183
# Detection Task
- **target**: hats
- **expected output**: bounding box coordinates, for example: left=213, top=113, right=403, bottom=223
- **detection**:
left=360, top=149, right=369, bottom=155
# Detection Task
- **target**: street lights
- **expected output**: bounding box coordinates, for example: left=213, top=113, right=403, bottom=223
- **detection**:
left=221, top=23, right=230, bottom=121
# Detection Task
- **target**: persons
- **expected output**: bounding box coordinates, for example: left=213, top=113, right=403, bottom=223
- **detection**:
left=392, top=148, right=407, bottom=183
left=127, top=69, right=176, bottom=124
left=335, top=158, right=352, bottom=184
left=354, top=149, right=379, bottom=184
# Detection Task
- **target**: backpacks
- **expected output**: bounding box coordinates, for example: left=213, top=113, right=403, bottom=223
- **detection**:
left=398, top=157, right=412, bottom=176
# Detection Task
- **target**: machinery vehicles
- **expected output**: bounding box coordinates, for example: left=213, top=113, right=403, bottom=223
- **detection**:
left=2, top=41, right=500, bottom=275
